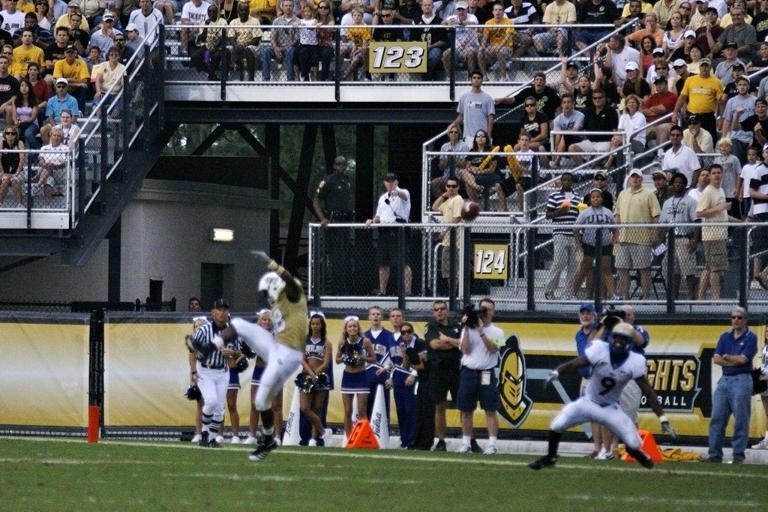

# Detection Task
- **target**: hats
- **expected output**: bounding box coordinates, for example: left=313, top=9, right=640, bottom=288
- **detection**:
left=706, top=7, right=719, bottom=18
left=56, top=77, right=68, bottom=84
left=733, top=63, right=744, bottom=72
left=688, top=115, right=700, bottom=124
left=567, top=62, right=578, bottom=70
left=125, top=24, right=138, bottom=32
left=754, top=97, right=767, bottom=106
left=733, top=77, right=751, bottom=84
left=695, top=1, right=709, bottom=4
left=455, top=1, right=469, bottom=10
left=595, top=171, right=607, bottom=178
left=64, top=45, right=77, bottom=51
left=383, top=173, right=396, bottom=181
left=212, top=298, right=229, bottom=308
left=726, top=41, right=738, bottom=50
left=102, top=14, right=114, bottom=22
left=580, top=304, right=593, bottom=312
left=652, top=170, right=667, bottom=179
left=624, top=30, right=700, bottom=72
left=630, top=168, right=642, bottom=177
left=68, top=1, right=80, bottom=7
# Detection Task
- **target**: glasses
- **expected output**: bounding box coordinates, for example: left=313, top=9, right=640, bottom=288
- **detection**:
left=336, top=161, right=346, bottom=166
left=434, top=306, right=446, bottom=311
left=113, top=38, right=125, bottom=41
left=5, top=132, right=16, bottom=135
left=37, top=2, right=46, bottom=6
left=401, top=328, right=413, bottom=336
left=595, top=178, right=607, bottom=181
left=450, top=131, right=458, bottom=134
left=108, top=53, right=119, bottom=58
left=318, top=5, right=329, bottom=10
left=71, top=12, right=81, bottom=16
left=524, top=103, right=536, bottom=107
left=57, top=85, right=65, bottom=88
left=447, top=184, right=458, bottom=188
left=652, top=176, right=663, bottom=181
left=3, top=51, right=12, bottom=55
left=382, top=13, right=391, bottom=18
left=731, top=315, right=744, bottom=320
left=476, top=133, right=485, bottom=137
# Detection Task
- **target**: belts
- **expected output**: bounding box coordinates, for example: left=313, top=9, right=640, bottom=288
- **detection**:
left=201, top=364, right=224, bottom=370
left=671, top=234, right=692, bottom=239
left=723, top=371, right=741, bottom=376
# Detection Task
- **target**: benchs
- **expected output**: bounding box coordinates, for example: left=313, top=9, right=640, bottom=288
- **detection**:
left=163, top=23, right=188, bottom=61
left=23, top=103, right=120, bottom=173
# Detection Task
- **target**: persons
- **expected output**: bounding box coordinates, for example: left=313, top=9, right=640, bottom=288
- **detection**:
left=186, top=296, right=507, bottom=456
left=314, top=156, right=413, bottom=295
left=1, top=0, right=182, bottom=210
left=180, top=0, right=767, bottom=85
left=430, top=61, right=768, bottom=297
left=228, top=250, right=307, bottom=463
left=701, top=309, right=767, bottom=464
left=528, top=304, right=676, bottom=471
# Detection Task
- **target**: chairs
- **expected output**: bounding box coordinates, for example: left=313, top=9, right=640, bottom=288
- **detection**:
left=627, top=251, right=667, bottom=300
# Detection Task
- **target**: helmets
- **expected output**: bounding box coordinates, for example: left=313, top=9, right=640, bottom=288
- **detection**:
left=607, top=322, right=636, bottom=355
left=256, top=271, right=283, bottom=306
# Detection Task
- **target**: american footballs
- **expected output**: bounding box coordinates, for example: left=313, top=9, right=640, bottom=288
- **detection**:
left=461, top=202, right=479, bottom=221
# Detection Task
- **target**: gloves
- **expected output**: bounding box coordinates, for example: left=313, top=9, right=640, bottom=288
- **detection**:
left=660, top=418, right=678, bottom=439
left=544, top=373, right=559, bottom=389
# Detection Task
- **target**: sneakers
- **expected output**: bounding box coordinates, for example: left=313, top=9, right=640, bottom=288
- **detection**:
left=185, top=334, right=209, bottom=362
left=180, top=430, right=498, bottom=462
left=584, top=446, right=619, bottom=461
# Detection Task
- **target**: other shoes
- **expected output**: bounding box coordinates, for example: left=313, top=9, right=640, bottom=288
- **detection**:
left=733, top=459, right=743, bottom=466
left=700, top=458, right=722, bottom=466
left=625, top=446, right=654, bottom=468
left=750, top=438, right=767, bottom=449
left=528, top=455, right=561, bottom=469
